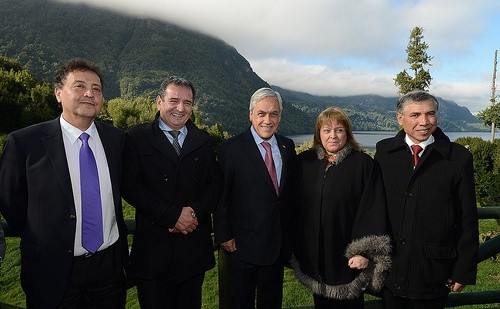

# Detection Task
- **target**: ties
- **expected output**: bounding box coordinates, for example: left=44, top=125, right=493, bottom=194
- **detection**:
left=410, top=143, right=424, bottom=173
left=79, top=133, right=104, bottom=255
left=260, top=141, right=279, bottom=195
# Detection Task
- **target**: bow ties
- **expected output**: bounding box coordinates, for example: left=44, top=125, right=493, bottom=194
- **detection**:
left=168, top=129, right=182, bottom=161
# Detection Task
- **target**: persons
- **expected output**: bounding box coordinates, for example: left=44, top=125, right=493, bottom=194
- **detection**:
left=122, top=76, right=223, bottom=309
left=290, top=107, right=392, bottom=309
left=0, top=59, right=128, bottom=309
left=374, top=90, right=479, bottom=309
left=213, top=87, right=297, bottom=309
